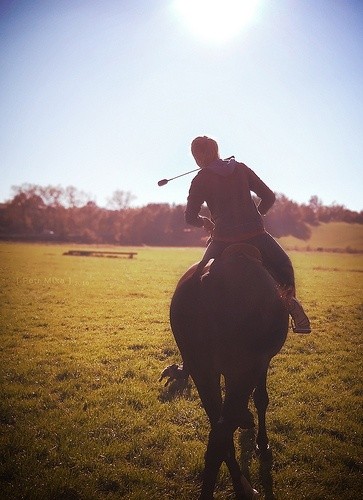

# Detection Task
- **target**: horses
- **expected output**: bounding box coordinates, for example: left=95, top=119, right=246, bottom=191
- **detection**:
left=169, top=242, right=289, bottom=500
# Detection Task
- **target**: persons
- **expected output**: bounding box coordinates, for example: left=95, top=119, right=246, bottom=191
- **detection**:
left=177, top=136, right=312, bottom=334
